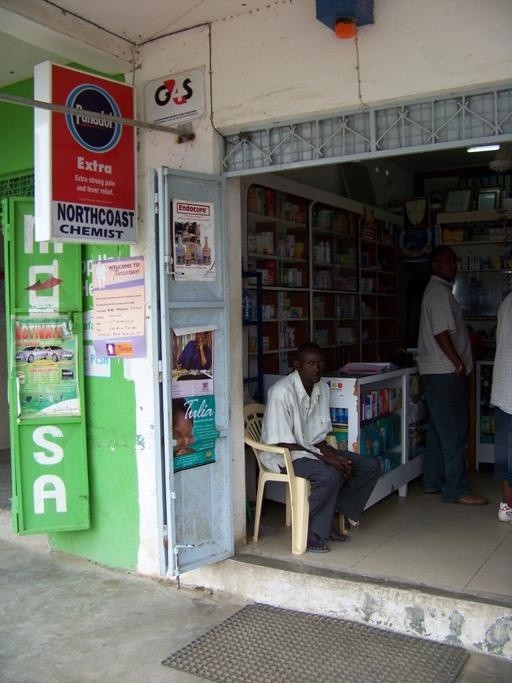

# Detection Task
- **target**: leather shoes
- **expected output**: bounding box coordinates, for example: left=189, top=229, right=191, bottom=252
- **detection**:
left=326, top=528, right=347, bottom=542
left=449, top=494, right=489, bottom=505
left=307, top=541, right=329, bottom=553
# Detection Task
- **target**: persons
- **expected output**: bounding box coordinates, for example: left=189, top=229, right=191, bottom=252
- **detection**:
left=418, top=243, right=490, bottom=505
left=171, top=398, right=197, bottom=458
left=490, top=286, right=512, bottom=524
left=177, top=332, right=211, bottom=370
left=259, top=342, right=382, bottom=554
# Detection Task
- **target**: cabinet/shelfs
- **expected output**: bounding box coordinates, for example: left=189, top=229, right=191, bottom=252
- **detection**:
left=361, top=212, right=406, bottom=368
left=405, top=365, right=434, bottom=476
left=308, top=204, right=363, bottom=377
left=241, top=181, right=311, bottom=412
left=474, top=360, right=500, bottom=481
left=429, top=215, right=512, bottom=353
left=261, top=370, right=410, bottom=512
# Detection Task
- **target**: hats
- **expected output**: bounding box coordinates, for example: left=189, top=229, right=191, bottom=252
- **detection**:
left=427, top=244, right=463, bottom=263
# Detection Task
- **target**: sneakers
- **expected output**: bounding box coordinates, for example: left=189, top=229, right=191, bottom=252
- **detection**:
left=497, top=501, right=512, bottom=522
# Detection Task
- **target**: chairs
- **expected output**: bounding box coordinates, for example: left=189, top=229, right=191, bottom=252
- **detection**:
left=239, top=405, right=352, bottom=553
